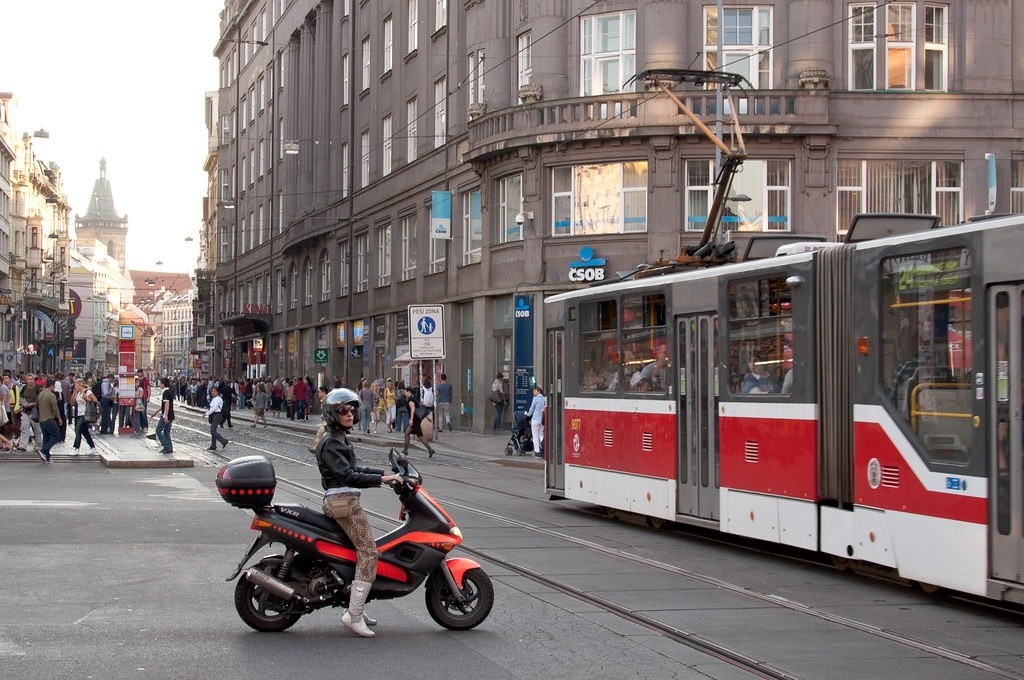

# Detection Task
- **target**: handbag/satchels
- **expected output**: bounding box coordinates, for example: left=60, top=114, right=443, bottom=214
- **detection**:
left=222, top=410, right=228, bottom=423
left=30, top=407, right=39, bottom=423
left=407, top=397, right=429, bottom=420
left=135, top=399, right=145, bottom=412
left=82, top=389, right=98, bottom=423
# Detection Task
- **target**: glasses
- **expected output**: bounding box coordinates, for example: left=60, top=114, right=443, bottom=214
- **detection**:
left=334, top=405, right=356, bottom=416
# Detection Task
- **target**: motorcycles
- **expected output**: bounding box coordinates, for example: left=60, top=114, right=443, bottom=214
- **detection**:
left=214, top=446, right=495, bottom=632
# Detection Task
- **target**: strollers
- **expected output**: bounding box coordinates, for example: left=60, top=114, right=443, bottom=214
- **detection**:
left=503, top=411, right=534, bottom=457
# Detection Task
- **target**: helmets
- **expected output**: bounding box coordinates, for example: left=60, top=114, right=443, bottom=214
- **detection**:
left=321, top=387, right=363, bottom=427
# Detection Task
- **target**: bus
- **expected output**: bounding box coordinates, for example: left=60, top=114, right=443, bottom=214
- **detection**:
left=546, top=68, right=1024, bottom=606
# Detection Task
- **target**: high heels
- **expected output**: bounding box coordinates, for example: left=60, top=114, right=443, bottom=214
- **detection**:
left=427, top=449, right=436, bottom=458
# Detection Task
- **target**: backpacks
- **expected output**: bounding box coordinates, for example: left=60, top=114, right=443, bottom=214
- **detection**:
left=91, top=380, right=108, bottom=402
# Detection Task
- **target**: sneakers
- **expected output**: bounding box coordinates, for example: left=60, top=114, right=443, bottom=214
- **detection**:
left=67, top=447, right=80, bottom=456
left=84, top=447, right=98, bottom=456
left=222, top=440, right=228, bottom=448
left=207, top=447, right=216, bottom=450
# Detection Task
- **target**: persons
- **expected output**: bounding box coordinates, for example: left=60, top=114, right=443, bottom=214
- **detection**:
left=421, top=374, right=454, bottom=433
left=490, top=371, right=508, bottom=431
left=582, top=340, right=667, bottom=392
left=147, top=378, right=176, bottom=453
left=307, top=387, right=404, bottom=636
left=886, top=331, right=947, bottom=407
left=742, top=343, right=794, bottom=393
left=525, top=385, right=545, bottom=457
left=355, top=377, right=408, bottom=436
left=0, top=369, right=120, bottom=465
left=307, top=386, right=330, bottom=453
left=202, top=387, right=228, bottom=450
left=237, top=375, right=316, bottom=427
left=169, top=375, right=240, bottom=428
left=333, top=375, right=351, bottom=389
left=120, top=368, right=151, bottom=433
left=402, top=386, right=436, bottom=458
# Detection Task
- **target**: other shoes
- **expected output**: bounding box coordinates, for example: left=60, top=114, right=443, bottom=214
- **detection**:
left=272, top=415, right=279, bottom=418
left=37, top=449, right=47, bottom=462
left=447, top=422, right=453, bottom=431
left=158, top=449, right=173, bottom=453
left=250, top=424, right=256, bottom=427
left=439, top=430, right=442, bottom=432
left=34, top=445, right=43, bottom=451
left=286, top=414, right=309, bottom=422
left=228, top=425, right=233, bottom=427
left=220, top=424, right=224, bottom=428
left=532, top=452, right=540, bottom=457
left=367, top=429, right=370, bottom=434
left=42, top=459, right=53, bottom=464
left=17, top=445, right=27, bottom=451
left=263, top=423, right=269, bottom=428
left=387, top=429, right=392, bottom=433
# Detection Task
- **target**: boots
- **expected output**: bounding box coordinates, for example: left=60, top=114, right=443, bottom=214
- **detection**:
left=340, top=579, right=378, bottom=638
